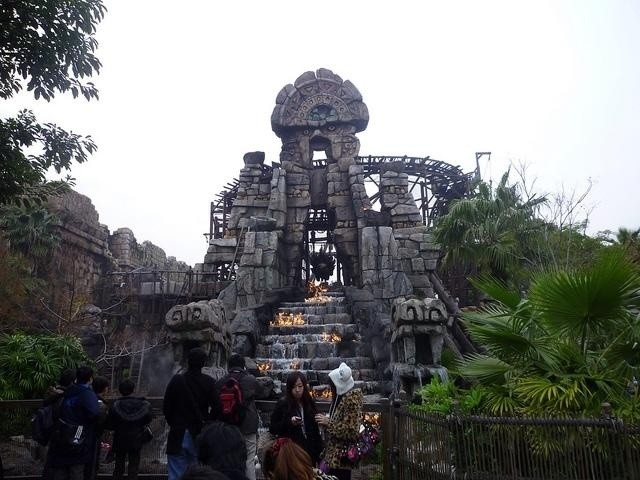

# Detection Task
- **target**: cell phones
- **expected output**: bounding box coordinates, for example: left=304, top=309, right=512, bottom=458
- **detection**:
left=295, top=416, right=302, bottom=421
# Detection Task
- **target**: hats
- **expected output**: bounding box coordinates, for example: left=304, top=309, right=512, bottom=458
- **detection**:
left=328, top=362, right=354, bottom=396
left=227, top=354, right=245, bottom=370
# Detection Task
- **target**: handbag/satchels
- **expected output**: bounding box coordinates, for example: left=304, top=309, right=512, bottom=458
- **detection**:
left=144, top=425, right=154, bottom=444
left=98, top=441, right=116, bottom=466
left=344, top=418, right=382, bottom=467
left=54, top=416, right=89, bottom=457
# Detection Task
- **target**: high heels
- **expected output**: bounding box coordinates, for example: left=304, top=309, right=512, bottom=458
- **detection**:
left=30, top=392, right=63, bottom=447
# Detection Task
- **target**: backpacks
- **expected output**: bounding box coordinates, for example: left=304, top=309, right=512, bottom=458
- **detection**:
left=215, top=371, right=248, bottom=426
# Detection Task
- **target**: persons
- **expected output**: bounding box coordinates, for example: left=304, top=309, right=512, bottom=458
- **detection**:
left=162, top=349, right=263, bottom=480
left=41, top=366, right=152, bottom=480
left=262, top=362, right=363, bottom=480
